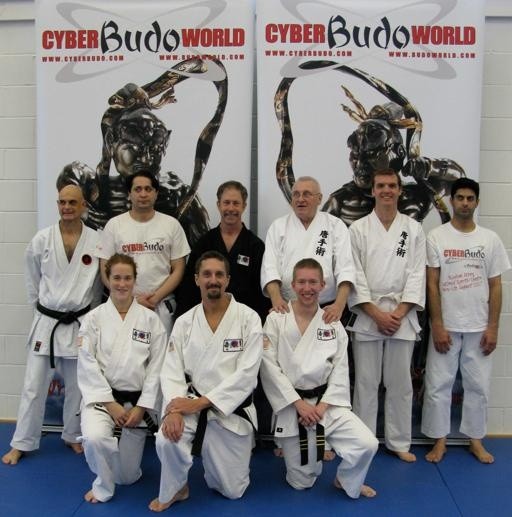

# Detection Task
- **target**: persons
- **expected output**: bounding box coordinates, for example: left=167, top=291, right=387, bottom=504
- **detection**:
left=96, top=168, right=192, bottom=333
left=347, top=165, right=427, bottom=462
left=176, top=178, right=273, bottom=454
left=147, top=246, right=263, bottom=512
left=2, top=180, right=104, bottom=467
left=260, top=174, right=355, bottom=323
left=76, top=250, right=170, bottom=508
left=56, top=81, right=211, bottom=247
left=256, top=255, right=381, bottom=498
left=320, top=83, right=465, bottom=225
left=419, top=176, right=512, bottom=464
